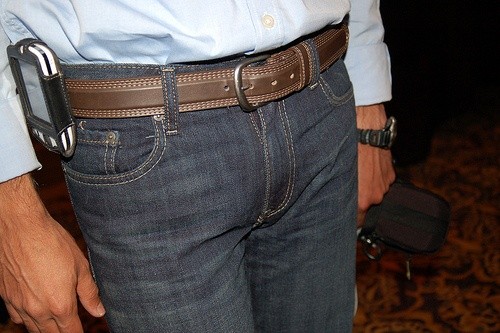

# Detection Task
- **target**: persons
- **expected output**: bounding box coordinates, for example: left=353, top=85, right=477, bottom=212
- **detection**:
left=0, top=0, right=398, bottom=333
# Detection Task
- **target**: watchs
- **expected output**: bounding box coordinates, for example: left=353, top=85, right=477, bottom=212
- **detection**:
left=343, top=113, right=399, bottom=149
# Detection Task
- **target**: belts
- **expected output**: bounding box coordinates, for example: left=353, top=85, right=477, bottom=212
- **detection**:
left=61, top=22, right=348, bottom=120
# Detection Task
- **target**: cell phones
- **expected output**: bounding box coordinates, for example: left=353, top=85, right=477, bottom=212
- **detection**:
left=6, top=38, right=72, bottom=154
